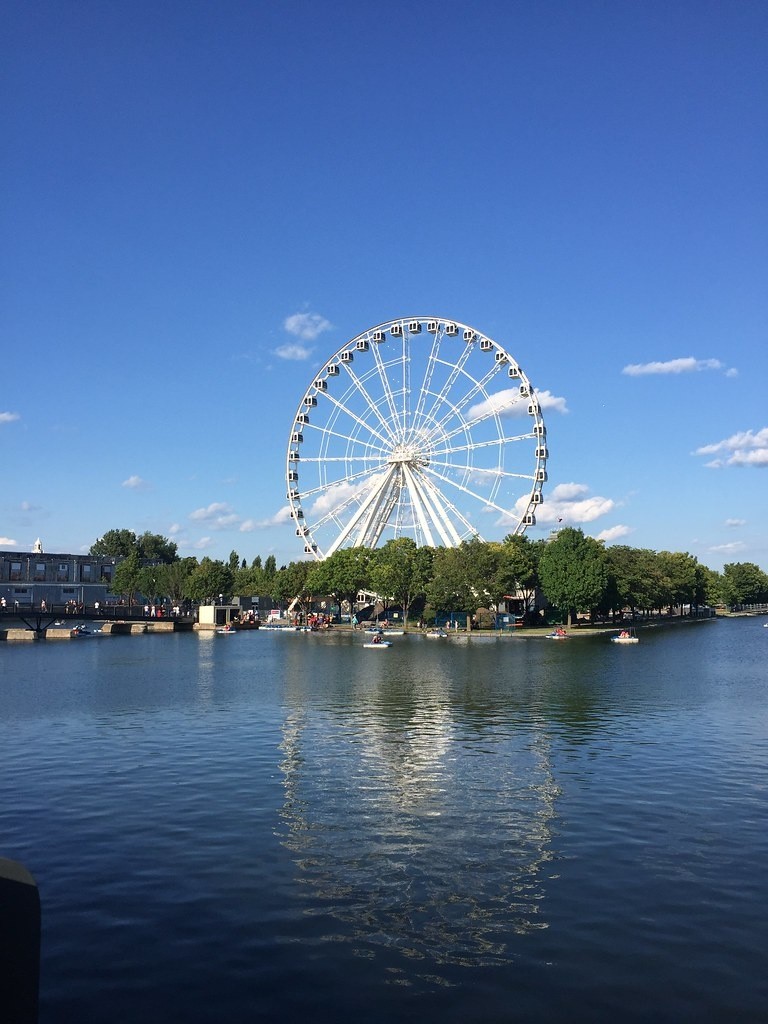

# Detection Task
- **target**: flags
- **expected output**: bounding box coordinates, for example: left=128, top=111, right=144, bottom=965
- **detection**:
left=556, top=515, right=563, bottom=524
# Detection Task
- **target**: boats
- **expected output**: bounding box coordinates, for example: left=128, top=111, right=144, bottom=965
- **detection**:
left=94, top=629, right=103, bottom=633
left=364, top=627, right=381, bottom=635
left=362, top=641, right=395, bottom=649
left=763, top=623, right=768, bottom=628
left=217, top=629, right=236, bottom=634
left=55, top=622, right=60, bottom=626
left=380, top=629, right=404, bottom=636
left=258, top=625, right=300, bottom=632
left=426, top=632, right=449, bottom=638
left=545, top=632, right=569, bottom=639
left=609, top=636, right=640, bottom=644
left=300, top=626, right=313, bottom=633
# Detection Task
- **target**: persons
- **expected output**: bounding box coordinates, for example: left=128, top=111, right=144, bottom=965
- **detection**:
left=144, top=605, right=187, bottom=617
left=620, top=630, right=628, bottom=637
left=372, top=636, right=381, bottom=643
left=1, top=597, right=103, bottom=614
left=558, top=627, right=566, bottom=635
left=381, top=619, right=388, bottom=629
left=417, top=620, right=459, bottom=632
left=352, top=616, right=358, bottom=630
left=220, top=611, right=258, bottom=631
left=294, top=613, right=333, bottom=627
